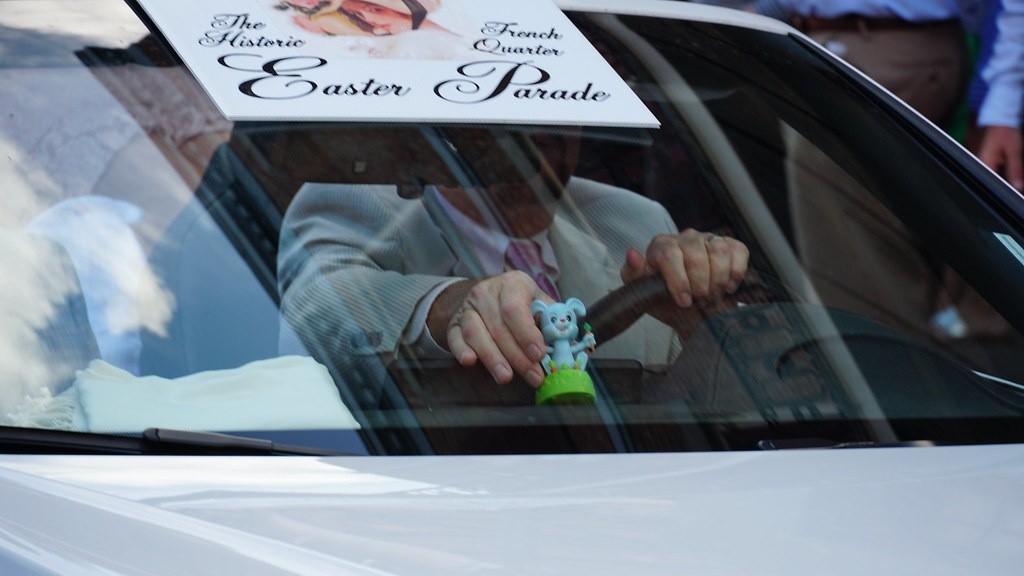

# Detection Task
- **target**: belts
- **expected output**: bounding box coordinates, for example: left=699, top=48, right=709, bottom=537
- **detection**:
left=790, top=13, right=952, bottom=32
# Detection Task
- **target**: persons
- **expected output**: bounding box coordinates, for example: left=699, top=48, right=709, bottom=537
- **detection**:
left=742, top=0, right=1024, bottom=346
left=277, top=125, right=749, bottom=386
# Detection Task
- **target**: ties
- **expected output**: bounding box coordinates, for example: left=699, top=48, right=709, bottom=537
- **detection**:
left=502, top=240, right=567, bottom=366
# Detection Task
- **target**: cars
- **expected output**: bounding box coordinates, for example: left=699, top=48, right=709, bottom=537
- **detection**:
left=0, top=0, right=1021, bottom=570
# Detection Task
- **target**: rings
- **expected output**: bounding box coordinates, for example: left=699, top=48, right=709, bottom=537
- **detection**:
left=706, top=235, right=726, bottom=244
